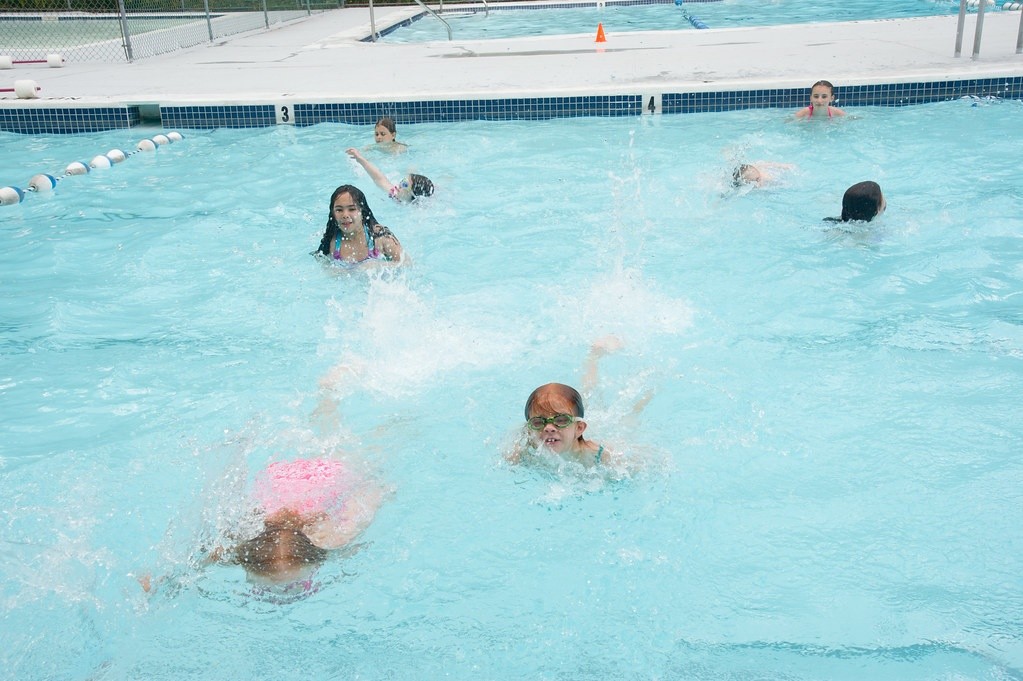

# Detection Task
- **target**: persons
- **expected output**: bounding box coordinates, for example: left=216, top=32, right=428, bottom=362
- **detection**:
left=734, top=163, right=766, bottom=186
left=796, top=80, right=847, bottom=116
left=345, top=148, right=434, bottom=203
left=840, top=181, right=887, bottom=224
left=317, top=184, right=402, bottom=261
left=374, top=118, right=396, bottom=146
left=524, top=383, right=612, bottom=465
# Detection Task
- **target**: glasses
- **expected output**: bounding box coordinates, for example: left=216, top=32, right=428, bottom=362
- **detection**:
left=401, top=178, right=415, bottom=199
left=527, top=413, right=585, bottom=431
